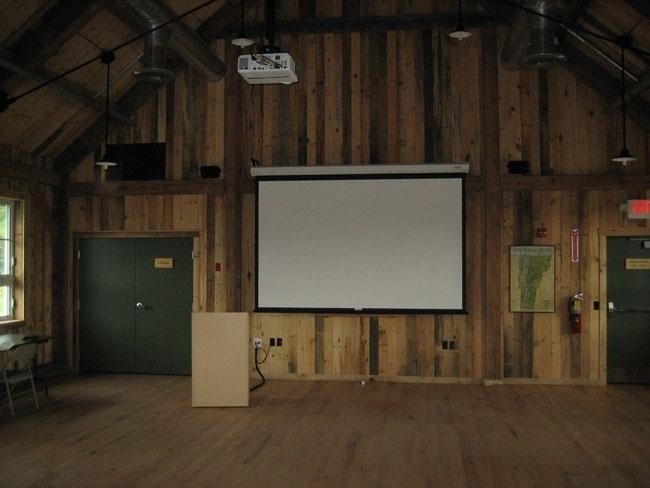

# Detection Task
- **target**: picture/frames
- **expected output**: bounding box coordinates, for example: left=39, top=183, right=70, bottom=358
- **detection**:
left=509, top=245, right=555, bottom=313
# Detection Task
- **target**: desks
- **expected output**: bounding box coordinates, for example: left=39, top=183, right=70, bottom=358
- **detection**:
left=0, top=331, right=51, bottom=399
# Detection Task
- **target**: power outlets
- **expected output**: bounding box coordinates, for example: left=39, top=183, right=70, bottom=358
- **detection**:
left=253, top=339, right=262, bottom=348
left=442, top=340, right=456, bottom=351
left=270, top=338, right=282, bottom=346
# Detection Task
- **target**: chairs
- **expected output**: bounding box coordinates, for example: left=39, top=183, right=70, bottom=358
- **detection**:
left=1, top=343, right=39, bottom=417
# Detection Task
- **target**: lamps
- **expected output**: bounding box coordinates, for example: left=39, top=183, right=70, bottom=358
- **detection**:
left=612, top=44, right=637, bottom=166
left=448, top=0, right=472, bottom=40
left=230, top=0, right=256, bottom=48
left=94, top=50, right=117, bottom=170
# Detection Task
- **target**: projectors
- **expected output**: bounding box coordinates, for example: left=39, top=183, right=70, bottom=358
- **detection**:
left=238, top=56, right=299, bottom=87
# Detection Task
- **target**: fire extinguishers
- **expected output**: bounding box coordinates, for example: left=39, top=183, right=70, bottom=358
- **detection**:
left=569, top=294, right=584, bottom=334
